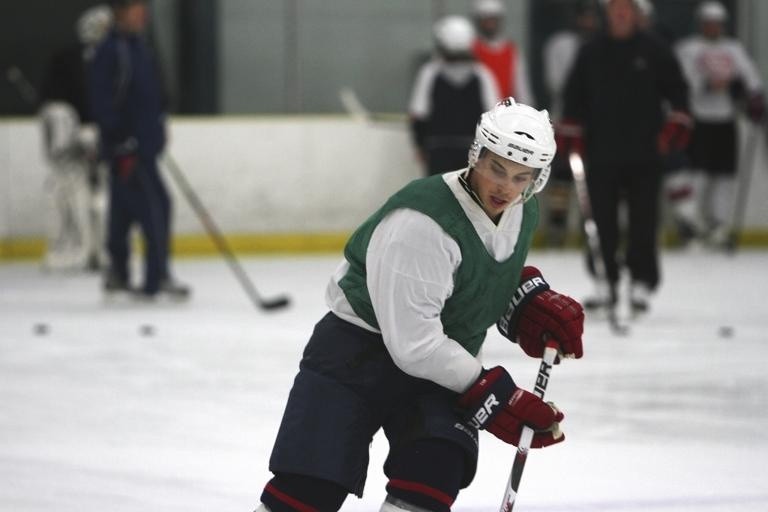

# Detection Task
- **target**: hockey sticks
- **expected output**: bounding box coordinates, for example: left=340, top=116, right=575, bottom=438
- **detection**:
left=564, top=125, right=632, bottom=334
left=164, top=153, right=288, bottom=310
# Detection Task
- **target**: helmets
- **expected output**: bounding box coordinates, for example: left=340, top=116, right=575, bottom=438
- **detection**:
left=468, top=95, right=557, bottom=210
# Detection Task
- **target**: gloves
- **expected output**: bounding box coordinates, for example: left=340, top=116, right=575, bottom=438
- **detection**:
left=496, top=265, right=585, bottom=360
left=455, top=365, right=565, bottom=448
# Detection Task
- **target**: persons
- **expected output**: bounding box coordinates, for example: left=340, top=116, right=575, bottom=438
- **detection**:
left=254, top=95, right=585, bottom=512
